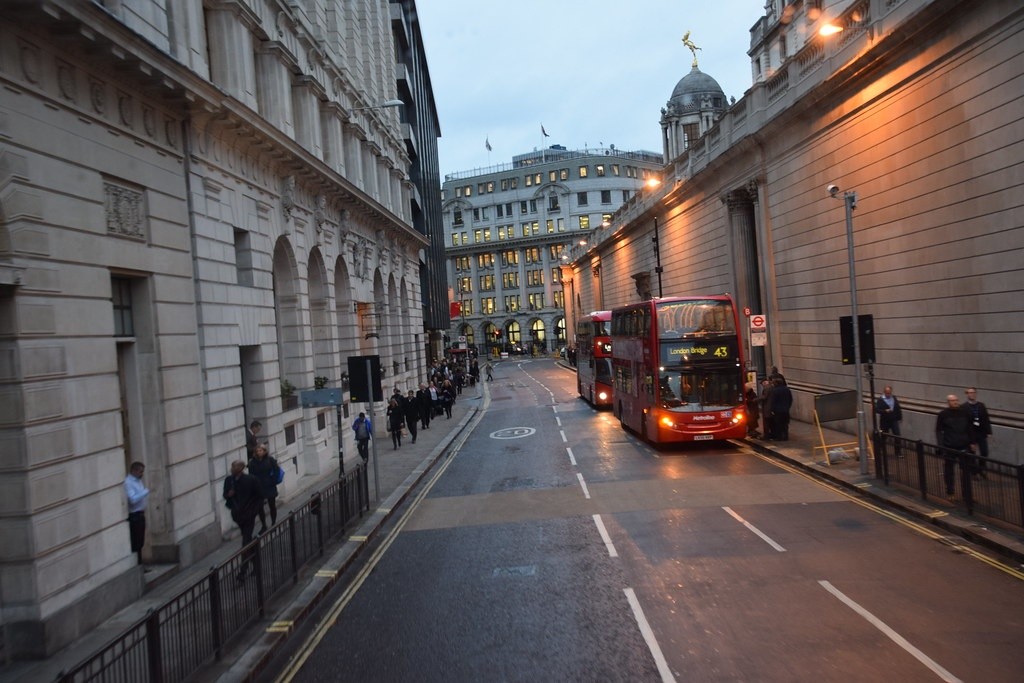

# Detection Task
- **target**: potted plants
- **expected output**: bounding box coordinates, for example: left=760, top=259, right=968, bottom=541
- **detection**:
left=280, top=379, right=299, bottom=411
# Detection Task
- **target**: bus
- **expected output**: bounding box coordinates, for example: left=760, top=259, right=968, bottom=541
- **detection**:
left=574, top=292, right=750, bottom=449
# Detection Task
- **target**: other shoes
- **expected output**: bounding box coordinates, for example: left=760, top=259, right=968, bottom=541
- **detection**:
left=258, top=528, right=268, bottom=535
left=412, top=439, right=416, bottom=443
left=237, top=574, right=245, bottom=586
left=896, top=453, right=904, bottom=457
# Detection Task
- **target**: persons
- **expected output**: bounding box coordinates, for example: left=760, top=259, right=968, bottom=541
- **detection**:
left=745, top=366, right=793, bottom=441
left=247, top=443, right=279, bottom=535
left=565, top=346, right=577, bottom=366
left=665, top=374, right=681, bottom=402
left=682, top=39, right=702, bottom=56
left=875, top=386, right=904, bottom=458
left=387, top=334, right=480, bottom=450
left=492, top=344, right=499, bottom=356
left=935, top=387, right=993, bottom=511
left=352, top=412, right=372, bottom=461
left=222, top=460, right=259, bottom=578
left=125, top=462, right=154, bottom=573
left=485, top=362, right=494, bottom=382
left=529, top=338, right=547, bottom=358
left=246, top=421, right=262, bottom=460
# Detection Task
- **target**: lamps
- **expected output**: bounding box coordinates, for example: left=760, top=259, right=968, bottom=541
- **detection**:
left=819, top=24, right=874, bottom=40
left=349, top=99, right=405, bottom=111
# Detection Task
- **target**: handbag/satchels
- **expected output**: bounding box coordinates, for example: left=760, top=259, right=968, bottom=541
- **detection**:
left=270, top=466, right=284, bottom=483
left=387, top=419, right=392, bottom=431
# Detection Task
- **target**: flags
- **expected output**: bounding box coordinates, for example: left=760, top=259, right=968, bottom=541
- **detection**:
left=451, top=302, right=462, bottom=318
left=541, top=125, right=549, bottom=136
left=486, top=137, right=492, bottom=151
